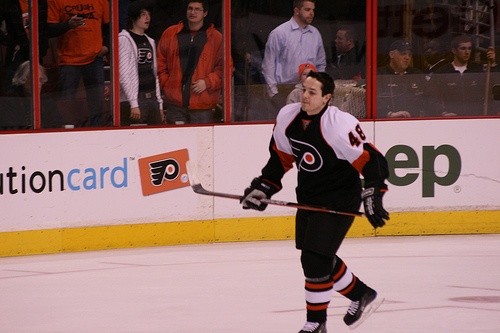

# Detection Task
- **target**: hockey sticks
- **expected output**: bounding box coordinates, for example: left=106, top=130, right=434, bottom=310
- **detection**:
left=186, top=159, right=368, bottom=218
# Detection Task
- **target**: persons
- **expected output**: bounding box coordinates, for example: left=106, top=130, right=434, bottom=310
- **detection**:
left=328, top=24, right=364, bottom=80
left=286, top=62, right=318, bottom=105
left=426, top=34, right=500, bottom=117
left=117, top=3, right=165, bottom=126
left=377, top=39, right=425, bottom=118
left=240, top=70, right=390, bottom=333
left=0, top=0, right=112, bottom=127
left=157, top=0, right=234, bottom=124
left=263, top=0, right=327, bottom=111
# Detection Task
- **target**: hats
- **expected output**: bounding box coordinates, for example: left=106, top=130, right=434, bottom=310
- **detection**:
left=390, top=40, right=414, bottom=51
left=298, top=63, right=317, bottom=81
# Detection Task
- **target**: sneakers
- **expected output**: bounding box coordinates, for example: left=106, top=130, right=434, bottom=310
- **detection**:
left=342, top=286, right=386, bottom=330
left=298, top=321, right=327, bottom=333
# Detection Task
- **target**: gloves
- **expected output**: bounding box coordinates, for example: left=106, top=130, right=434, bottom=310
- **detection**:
left=240, top=175, right=283, bottom=212
left=360, top=182, right=390, bottom=229
